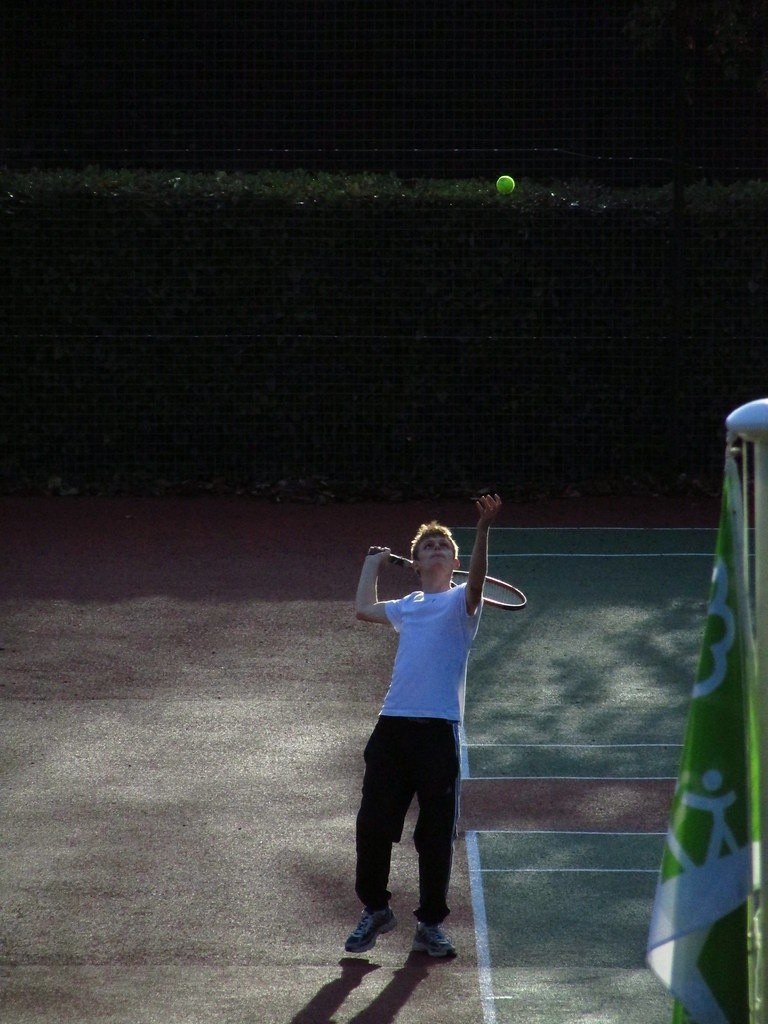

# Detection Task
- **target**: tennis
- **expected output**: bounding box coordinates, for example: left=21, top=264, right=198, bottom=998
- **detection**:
left=495, top=174, right=516, bottom=196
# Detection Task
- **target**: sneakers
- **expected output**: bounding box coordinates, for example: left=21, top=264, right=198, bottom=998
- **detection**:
left=346, top=905, right=397, bottom=953
left=413, top=922, right=456, bottom=958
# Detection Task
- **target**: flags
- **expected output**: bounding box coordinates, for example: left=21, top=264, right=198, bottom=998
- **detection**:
left=642, top=455, right=767, bottom=1023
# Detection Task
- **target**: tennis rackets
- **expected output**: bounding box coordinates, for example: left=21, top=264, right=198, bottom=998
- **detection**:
left=367, top=546, right=528, bottom=611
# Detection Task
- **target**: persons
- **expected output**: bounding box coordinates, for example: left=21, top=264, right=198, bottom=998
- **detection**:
left=344, top=492, right=503, bottom=957
